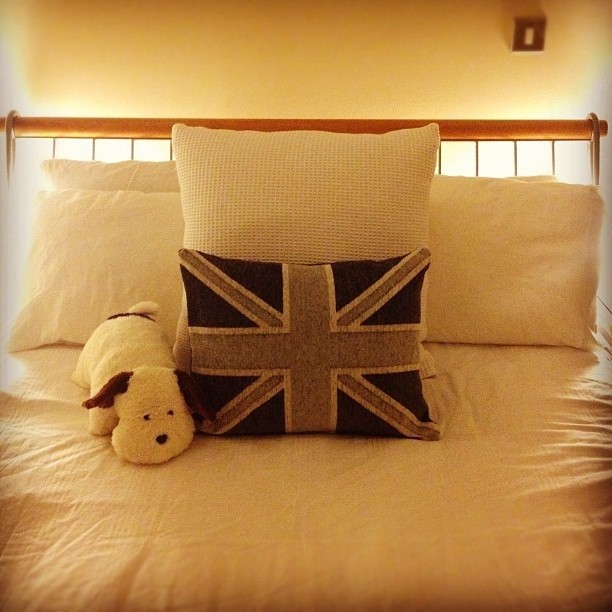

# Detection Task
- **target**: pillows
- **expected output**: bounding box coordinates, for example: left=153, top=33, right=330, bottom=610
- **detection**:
left=172, top=123, right=441, bottom=382
left=8, top=188, right=185, bottom=355
left=178, top=248, right=441, bottom=442
left=41, top=157, right=179, bottom=193
left=427, top=175, right=611, bottom=358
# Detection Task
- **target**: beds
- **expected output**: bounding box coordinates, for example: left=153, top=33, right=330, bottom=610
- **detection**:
left=0, top=110, right=611, bottom=610
